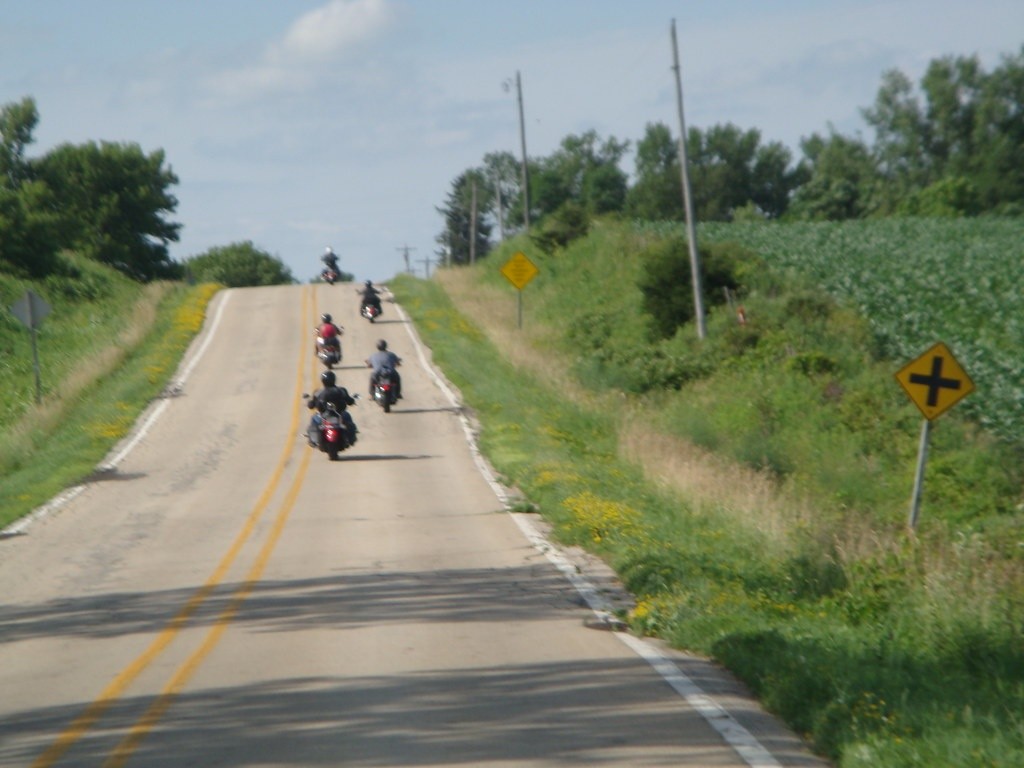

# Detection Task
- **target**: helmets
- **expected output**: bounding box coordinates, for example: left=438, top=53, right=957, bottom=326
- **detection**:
left=325, top=247, right=332, bottom=253
left=365, top=281, right=372, bottom=287
left=320, top=371, right=336, bottom=385
left=321, top=313, right=332, bottom=323
left=376, top=339, right=387, bottom=350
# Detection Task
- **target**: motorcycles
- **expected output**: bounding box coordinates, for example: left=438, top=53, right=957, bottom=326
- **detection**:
left=315, top=326, right=343, bottom=369
left=358, top=288, right=379, bottom=322
left=322, top=263, right=338, bottom=284
left=302, top=391, right=358, bottom=459
left=366, top=358, right=402, bottom=412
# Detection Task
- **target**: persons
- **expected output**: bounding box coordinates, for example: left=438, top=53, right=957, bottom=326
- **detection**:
left=358, top=280, right=384, bottom=319
left=307, top=370, right=358, bottom=448
left=365, top=340, right=404, bottom=403
left=313, top=314, right=342, bottom=363
left=320, top=246, right=341, bottom=277
